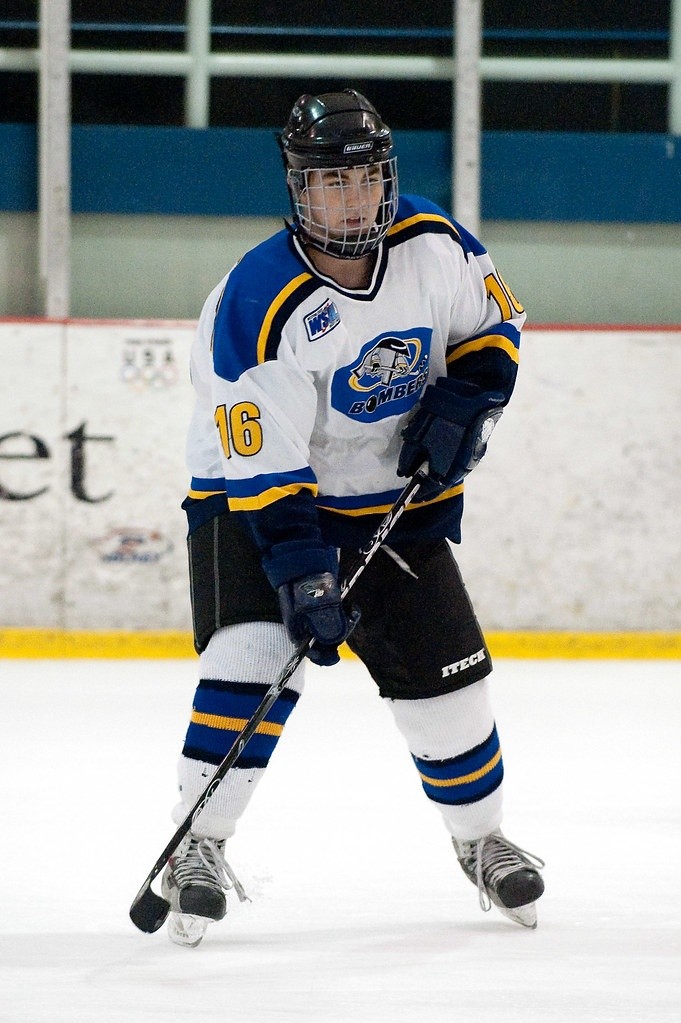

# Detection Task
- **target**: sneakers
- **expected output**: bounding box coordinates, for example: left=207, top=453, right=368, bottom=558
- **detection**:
left=161, top=824, right=252, bottom=949
left=451, top=827, right=546, bottom=930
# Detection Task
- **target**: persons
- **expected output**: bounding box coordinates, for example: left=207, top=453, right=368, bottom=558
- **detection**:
left=161, top=89, right=545, bottom=921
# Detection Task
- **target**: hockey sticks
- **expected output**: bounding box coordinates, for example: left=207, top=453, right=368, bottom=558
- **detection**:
left=126, top=458, right=432, bottom=935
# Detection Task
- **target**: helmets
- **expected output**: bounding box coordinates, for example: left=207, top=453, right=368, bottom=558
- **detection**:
left=274, top=88, right=400, bottom=260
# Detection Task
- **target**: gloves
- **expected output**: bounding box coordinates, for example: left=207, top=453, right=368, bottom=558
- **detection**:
left=278, top=572, right=362, bottom=667
left=396, top=376, right=506, bottom=504
